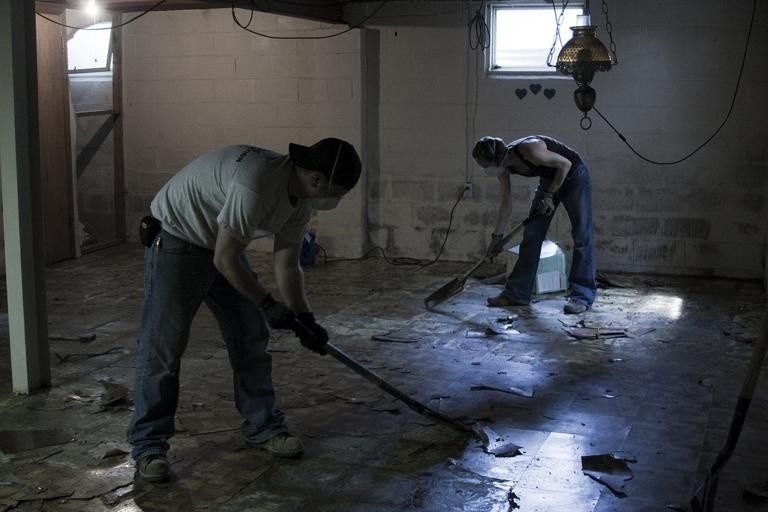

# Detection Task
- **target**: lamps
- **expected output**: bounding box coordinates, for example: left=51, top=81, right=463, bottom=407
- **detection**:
left=545, top=1, right=619, bottom=131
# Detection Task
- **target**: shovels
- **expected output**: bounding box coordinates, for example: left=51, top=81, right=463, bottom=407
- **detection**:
left=424, top=200, right=551, bottom=311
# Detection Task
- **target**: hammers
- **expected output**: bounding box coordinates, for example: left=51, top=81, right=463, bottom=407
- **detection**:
left=49, top=332, right=97, bottom=343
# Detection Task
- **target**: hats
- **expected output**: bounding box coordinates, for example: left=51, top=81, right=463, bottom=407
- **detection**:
left=287, top=133, right=362, bottom=191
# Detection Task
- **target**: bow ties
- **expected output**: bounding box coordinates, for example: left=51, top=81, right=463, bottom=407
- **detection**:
left=481, top=149, right=507, bottom=179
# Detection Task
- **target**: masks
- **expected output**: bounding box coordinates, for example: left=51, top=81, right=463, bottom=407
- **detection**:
left=305, top=175, right=339, bottom=211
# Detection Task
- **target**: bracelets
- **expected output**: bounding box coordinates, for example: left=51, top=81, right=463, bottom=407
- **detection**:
left=544, top=191, right=553, bottom=199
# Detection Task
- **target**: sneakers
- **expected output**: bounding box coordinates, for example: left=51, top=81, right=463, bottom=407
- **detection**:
left=486, top=291, right=531, bottom=306
left=561, top=297, right=593, bottom=314
left=244, top=426, right=306, bottom=458
left=134, top=455, right=174, bottom=481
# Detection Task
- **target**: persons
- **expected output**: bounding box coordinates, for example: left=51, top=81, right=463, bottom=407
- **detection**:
left=471, top=136, right=597, bottom=315
left=125, top=138, right=361, bottom=484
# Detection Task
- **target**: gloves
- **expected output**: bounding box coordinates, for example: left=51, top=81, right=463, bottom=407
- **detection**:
left=296, top=311, right=333, bottom=357
left=257, top=293, right=305, bottom=337
left=539, top=191, right=556, bottom=220
left=485, top=232, right=504, bottom=261
